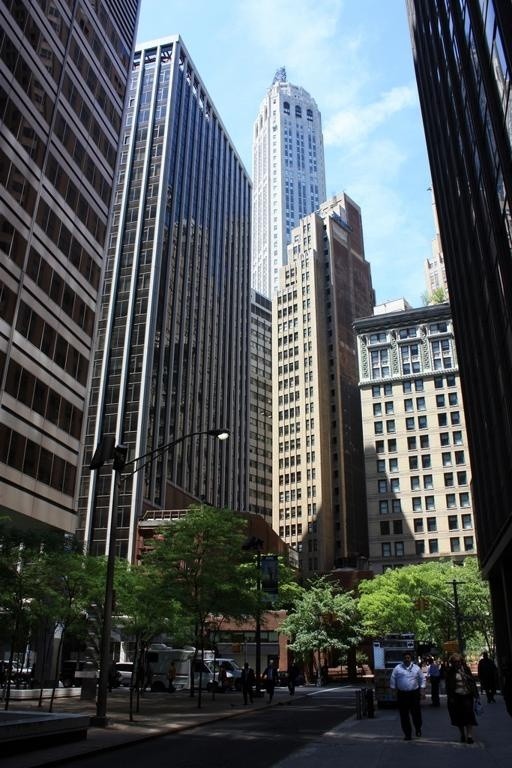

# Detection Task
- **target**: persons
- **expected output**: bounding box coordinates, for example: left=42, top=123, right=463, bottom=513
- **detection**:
left=439, top=654, right=479, bottom=744
left=427, top=658, right=441, bottom=707
left=478, top=652, right=501, bottom=704
left=108, top=651, right=300, bottom=706
left=389, top=652, right=427, bottom=741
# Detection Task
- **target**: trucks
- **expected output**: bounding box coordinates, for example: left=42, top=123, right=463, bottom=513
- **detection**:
left=371, top=631, right=420, bottom=710
left=136, top=642, right=235, bottom=693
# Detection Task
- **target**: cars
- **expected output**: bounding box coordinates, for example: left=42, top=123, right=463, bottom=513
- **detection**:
left=0, top=656, right=135, bottom=687
left=269, top=671, right=307, bottom=688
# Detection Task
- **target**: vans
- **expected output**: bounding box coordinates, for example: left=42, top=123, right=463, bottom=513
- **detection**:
left=207, top=659, right=247, bottom=691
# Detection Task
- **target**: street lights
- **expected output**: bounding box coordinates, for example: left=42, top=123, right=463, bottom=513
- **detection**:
left=240, top=532, right=267, bottom=695
left=90, top=426, right=232, bottom=727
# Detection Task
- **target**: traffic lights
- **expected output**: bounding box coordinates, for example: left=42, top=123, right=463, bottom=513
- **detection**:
left=413, top=598, right=420, bottom=610
left=424, top=598, right=429, bottom=610
